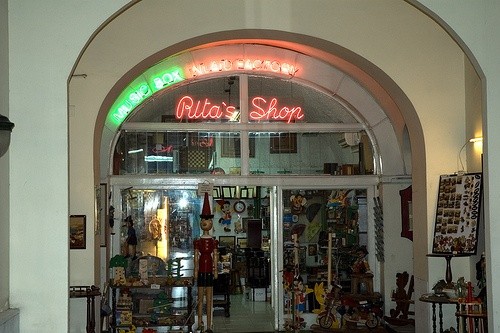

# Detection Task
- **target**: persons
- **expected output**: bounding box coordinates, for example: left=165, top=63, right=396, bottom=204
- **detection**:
left=193, top=191, right=219, bottom=333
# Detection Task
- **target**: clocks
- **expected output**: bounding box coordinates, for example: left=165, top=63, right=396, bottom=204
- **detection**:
left=233, top=200, right=246, bottom=214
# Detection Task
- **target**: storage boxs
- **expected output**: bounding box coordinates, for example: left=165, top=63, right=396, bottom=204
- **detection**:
left=157, top=316, right=173, bottom=325
left=240, top=278, right=246, bottom=286
left=217, top=261, right=230, bottom=274
left=120, top=311, right=133, bottom=325
left=250, top=287, right=267, bottom=302
left=175, top=315, right=184, bottom=324
left=221, top=255, right=232, bottom=269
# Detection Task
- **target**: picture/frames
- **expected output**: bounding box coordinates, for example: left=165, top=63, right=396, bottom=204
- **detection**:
left=240, top=188, right=248, bottom=199
left=247, top=188, right=254, bottom=199
left=222, top=186, right=231, bottom=199
left=308, top=243, right=317, bottom=256
left=100, top=183, right=107, bottom=247
left=242, top=217, right=254, bottom=233
left=219, top=236, right=235, bottom=252
left=269, top=133, right=297, bottom=154
left=213, top=186, right=221, bottom=199
left=237, top=238, right=248, bottom=248
left=212, top=189, right=219, bottom=199
left=70, top=215, right=86, bottom=250
left=230, top=186, right=236, bottom=199
left=221, top=137, right=255, bottom=159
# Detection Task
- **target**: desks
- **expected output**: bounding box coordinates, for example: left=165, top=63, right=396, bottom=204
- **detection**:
left=69, top=284, right=102, bottom=333
left=244, top=250, right=271, bottom=301
left=419, top=294, right=468, bottom=333
left=213, top=273, right=232, bottom=318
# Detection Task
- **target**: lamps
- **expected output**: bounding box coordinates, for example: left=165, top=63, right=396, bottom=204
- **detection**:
left=0, top=114, right=15, bottom=158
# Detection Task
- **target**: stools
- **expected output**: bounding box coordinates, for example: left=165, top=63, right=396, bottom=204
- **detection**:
left=350, top=273, right=374, bottom=320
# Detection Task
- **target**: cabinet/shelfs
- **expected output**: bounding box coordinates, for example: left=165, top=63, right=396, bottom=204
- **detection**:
left=321, top=189, right=368, bottom=274
left=108, top=276, right=195, bottom=333
